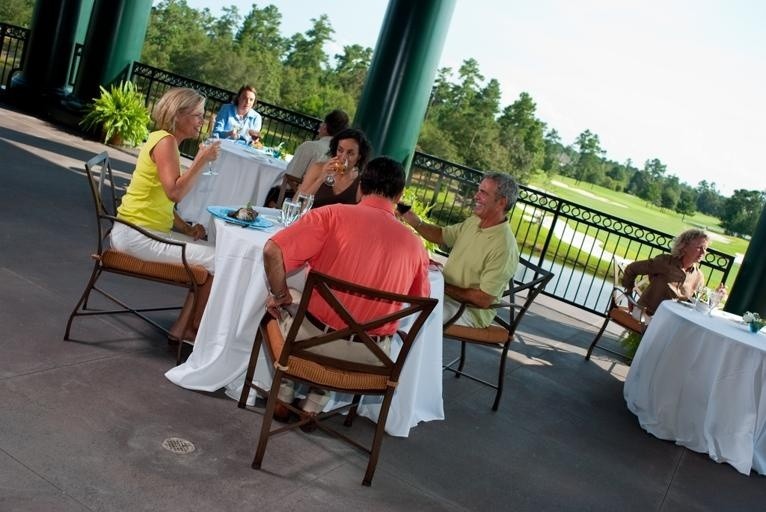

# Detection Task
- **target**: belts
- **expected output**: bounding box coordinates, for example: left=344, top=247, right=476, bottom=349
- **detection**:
left=305, top=310, right=386, bottom=343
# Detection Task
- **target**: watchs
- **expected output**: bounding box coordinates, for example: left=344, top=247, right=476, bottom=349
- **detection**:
left=269, top=289, right=288, bottom=299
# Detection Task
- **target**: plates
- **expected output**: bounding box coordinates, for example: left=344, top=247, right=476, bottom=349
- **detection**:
left=206, top=207, right=273, bottom=229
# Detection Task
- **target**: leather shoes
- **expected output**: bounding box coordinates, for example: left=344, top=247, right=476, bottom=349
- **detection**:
left=263, top=396, right=290, bottom=422
left=297, top=405, right=317, bottom=433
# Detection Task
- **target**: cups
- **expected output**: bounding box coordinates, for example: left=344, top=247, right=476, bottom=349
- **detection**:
left=297, top=192, right=315, bottom=216
left=280, top=198, right=301, bottom=226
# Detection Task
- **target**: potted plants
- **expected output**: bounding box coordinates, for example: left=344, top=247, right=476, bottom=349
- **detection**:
left=74, top=79, right=150, bottom=150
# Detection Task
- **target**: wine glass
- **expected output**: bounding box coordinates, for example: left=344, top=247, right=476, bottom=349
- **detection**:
left=250, top=131, right=259, bottom=145
left=706, top=289, right=723, bottom=319
left=323, top=157, right=349, bottom=185
left=397, top=194, right=412, bottom=218
left=202, top=136, right=221, bottom=176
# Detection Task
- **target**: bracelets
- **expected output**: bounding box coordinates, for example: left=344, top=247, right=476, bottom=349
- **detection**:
left=182, top=223, right=189, bottom=234
left=415, top=220, right=422, bottom=231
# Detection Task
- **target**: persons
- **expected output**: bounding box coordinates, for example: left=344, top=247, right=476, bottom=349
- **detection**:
left=265, top=157, right=431, bottom=433
left=209, top=86, right=262, bottom=143
left=622, top=230, right=728, bottom=324
left=263, top=109, right=348, bottom=208
left=395, top=172, right=518, bottom=327
left=111, top=89, right=220, bottom=342
left=292, top=128, right=371, bottom=213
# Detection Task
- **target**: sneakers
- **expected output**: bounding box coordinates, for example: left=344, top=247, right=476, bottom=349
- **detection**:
left=182, top=329, right=195, bottom=346
left=168, top=324, right=182, bottom=341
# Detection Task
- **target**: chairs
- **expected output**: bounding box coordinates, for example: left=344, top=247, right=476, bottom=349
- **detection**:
left=435, top=248, right=555, bottom=412
left=236, top=269, right=439, bottom=487
left=64, top=150, right=199, bottom=365
left=585, top=255, right=656, bottom=364
left=276, top=173, right=303, bottom=210
